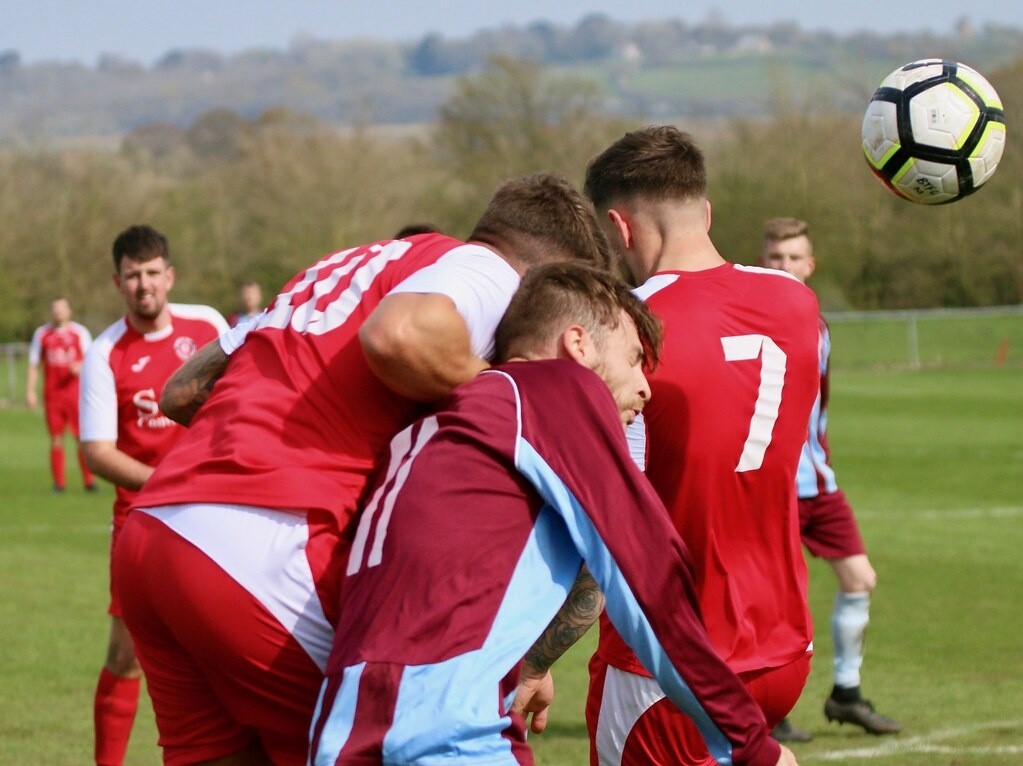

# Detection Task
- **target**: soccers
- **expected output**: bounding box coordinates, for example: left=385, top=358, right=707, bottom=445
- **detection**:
left=859, top=56, right=1008, bottom=208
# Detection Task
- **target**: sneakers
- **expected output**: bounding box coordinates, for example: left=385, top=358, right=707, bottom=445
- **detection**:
left=771, top=716, right=811, bottom=743
left=825, top=698, right=903, bottom=733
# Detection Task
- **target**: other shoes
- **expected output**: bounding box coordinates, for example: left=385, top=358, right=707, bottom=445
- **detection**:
left=84, top=484, right=98, bottom=492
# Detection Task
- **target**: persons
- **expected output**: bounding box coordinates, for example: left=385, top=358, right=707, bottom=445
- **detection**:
left=26, top=297, right=98, bottom=493
left=77, top=127, right=904, bottom=766
